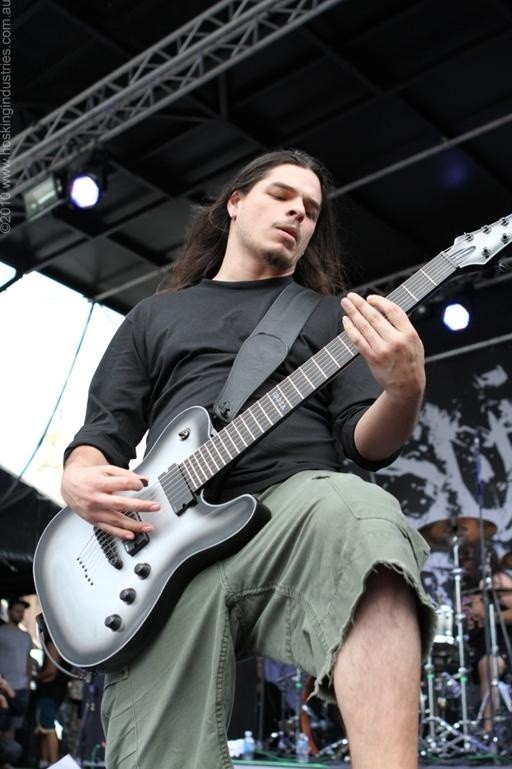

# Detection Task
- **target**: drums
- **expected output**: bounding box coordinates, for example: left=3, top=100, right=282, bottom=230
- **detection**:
left=301, top=673, right=439, bottom=763
left=429, top=603, right=469, bottom=671
left=439, top=680, right=511, bottom=756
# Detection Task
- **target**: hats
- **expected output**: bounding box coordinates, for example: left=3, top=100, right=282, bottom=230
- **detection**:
left=8, top=599, right=30, bottom=610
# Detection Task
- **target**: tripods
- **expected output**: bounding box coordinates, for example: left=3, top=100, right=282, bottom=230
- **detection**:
left=311, top=577, right=512, bottom=758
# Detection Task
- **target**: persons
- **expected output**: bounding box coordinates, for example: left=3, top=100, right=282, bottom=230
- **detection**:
left=465, top=541, right=512, bottom=733
left=59, top=147, right=439, bottom=769
left=0, top=597, right=92, bottom=769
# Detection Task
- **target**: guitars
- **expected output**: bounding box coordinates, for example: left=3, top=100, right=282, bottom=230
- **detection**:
left=33, top=216, right=509, bottom=673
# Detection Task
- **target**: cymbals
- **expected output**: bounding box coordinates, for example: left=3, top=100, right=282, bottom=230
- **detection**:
left=419, top=518, right=497, bottom=552
left=453, top=587, right=511, bottom=597
